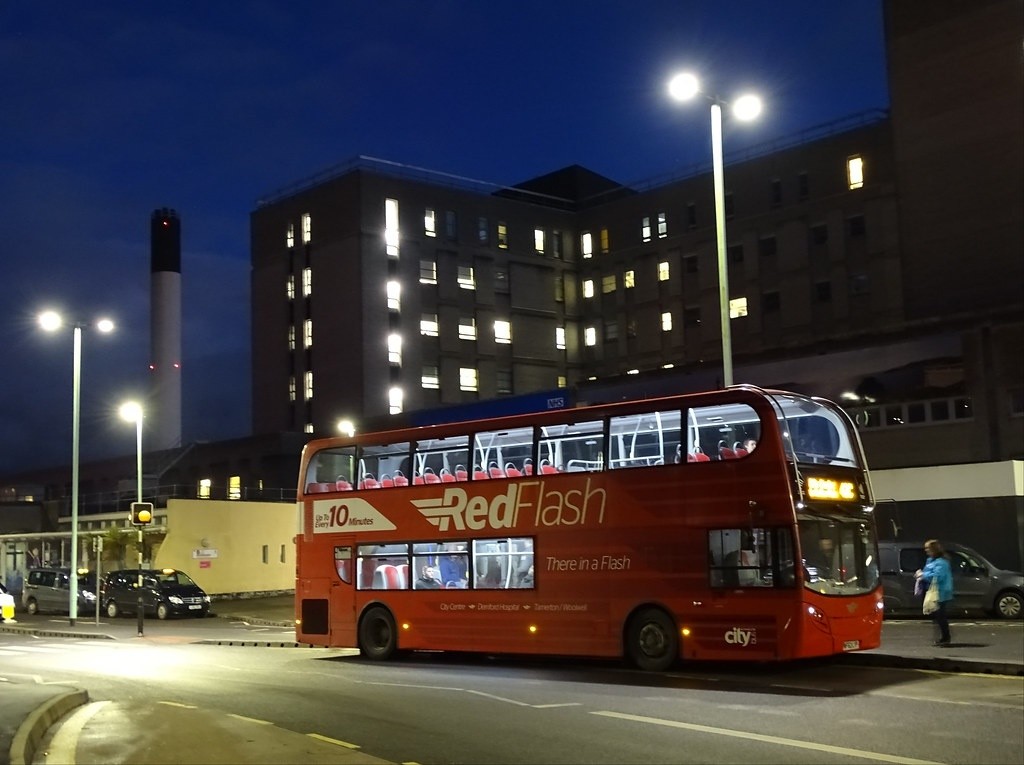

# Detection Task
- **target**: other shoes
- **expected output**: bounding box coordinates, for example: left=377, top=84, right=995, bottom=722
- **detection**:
left=931, top=639, right=950, bottom=647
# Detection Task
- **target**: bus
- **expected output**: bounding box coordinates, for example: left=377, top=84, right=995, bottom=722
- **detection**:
left=292, top=383, right=886, bottom=675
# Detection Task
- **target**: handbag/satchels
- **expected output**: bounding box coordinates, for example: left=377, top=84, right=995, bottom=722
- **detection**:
left=922, top=578, right=940, bottom=615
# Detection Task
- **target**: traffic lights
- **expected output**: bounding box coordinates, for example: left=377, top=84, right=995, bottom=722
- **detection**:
left=130, top=502, right=154, bottom=526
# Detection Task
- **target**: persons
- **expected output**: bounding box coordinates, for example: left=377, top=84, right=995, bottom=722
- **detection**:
left=914, top=540, right=954, bottom=647
left=817, top=539, right=836, bottom=575
left=416, top=537, right=533, bottom=589
left=521, top=458, right=533, bottom=475
left=744, top=437, right=757, bottom=453
left=675, top=440, right=744, bottom=464
left=742, top=530, right=767, bottom=578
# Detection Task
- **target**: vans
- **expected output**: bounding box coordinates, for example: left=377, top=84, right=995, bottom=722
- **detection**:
left=831, top=542, right=1024, bottom=620
left=102, top=568, right=211, bottom=619
left=21, top=567, right=107, bottom=617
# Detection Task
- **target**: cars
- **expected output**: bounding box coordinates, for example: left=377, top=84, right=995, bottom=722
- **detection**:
left=759, top=562, right=835, bottom=587
left=0, top=575, right=17, bottom=620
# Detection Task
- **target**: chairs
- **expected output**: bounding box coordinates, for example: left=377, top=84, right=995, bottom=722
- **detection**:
left=725, top=549, right=756, bottom=587
left=305, top=456, right=565, bottom=495
left=674, top=440, right=750, bottom=464
left=371, top=564, right=409, bottom=590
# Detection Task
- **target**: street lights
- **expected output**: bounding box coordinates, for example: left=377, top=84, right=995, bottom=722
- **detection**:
left=663, top=70, right=771, bottom=388
left=37, top=308, right=114, bottom=627
left=116, top=401, right=144, bottom=636
